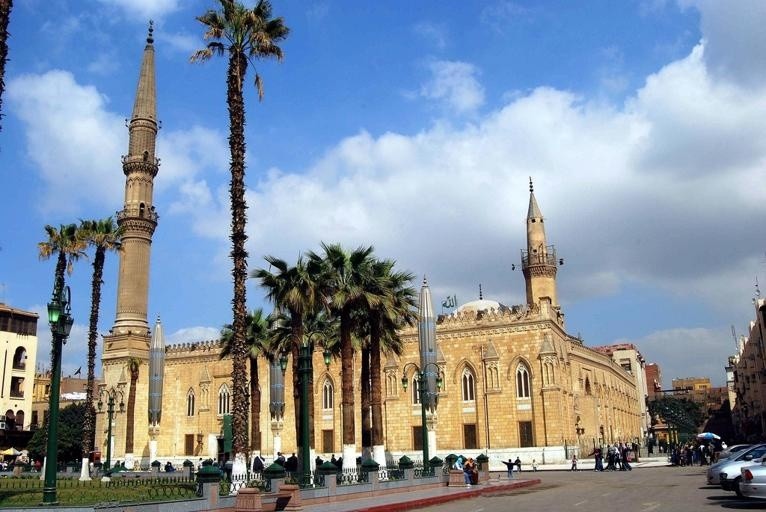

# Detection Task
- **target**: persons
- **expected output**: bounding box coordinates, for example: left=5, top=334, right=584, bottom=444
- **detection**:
left=87, top=453, right=126, bottom=478
left=212, top=457, right=219, bottom=467
left=514, top=456, right=522, bottom=474
left=356, top=456, right=362, bottom=465
left=530, top=458, right=540, bottom=472
left=277, top=451, right=343, bottom=472
left=452, top=457, right=473, bottom=487
left=587, top=438, right=716, bottom=472
left=500, top=459, right=522, bottom=477
left=570, top=455, right=578, bottom=472
left=197, top=457, right=203, bottom=470
left=169, top=462, right=176, bottom=472
left=463, top=457, right=478, bottom=485
left=164, top=461, right=170, bottom=472
left=0, top=453, right=40, bottom=475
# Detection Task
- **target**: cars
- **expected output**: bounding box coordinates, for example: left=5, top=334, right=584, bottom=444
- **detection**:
left=706, top=444, right=766, bottom=499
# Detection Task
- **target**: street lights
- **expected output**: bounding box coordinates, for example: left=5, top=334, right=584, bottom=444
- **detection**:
left=279, top=330, right=332, bottom=489
left=32, top=275, right=74, bottom=509
left=97, top=388, right=124, bottom=478
left=402, top=362, right=442, bottom=477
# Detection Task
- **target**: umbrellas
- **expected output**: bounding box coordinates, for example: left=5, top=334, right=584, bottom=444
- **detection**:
left=0, top=447, right=22, bottom=459
left=695, top=432, right=721, bottom=444
